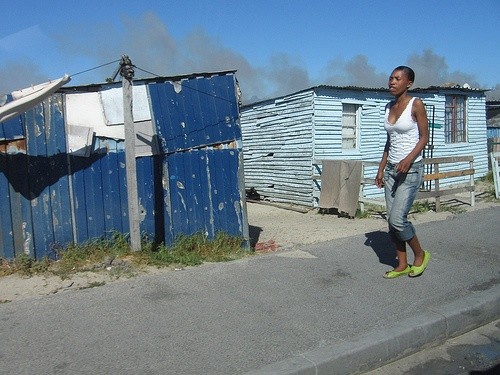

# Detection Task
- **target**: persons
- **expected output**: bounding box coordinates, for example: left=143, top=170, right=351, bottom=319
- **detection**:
left=376, top=66, right=431, bottom=278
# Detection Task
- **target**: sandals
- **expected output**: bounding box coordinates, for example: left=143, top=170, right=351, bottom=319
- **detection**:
left=383, top=264, right=410, bottom=279
left=408, top=249, right=430, bottom=277
